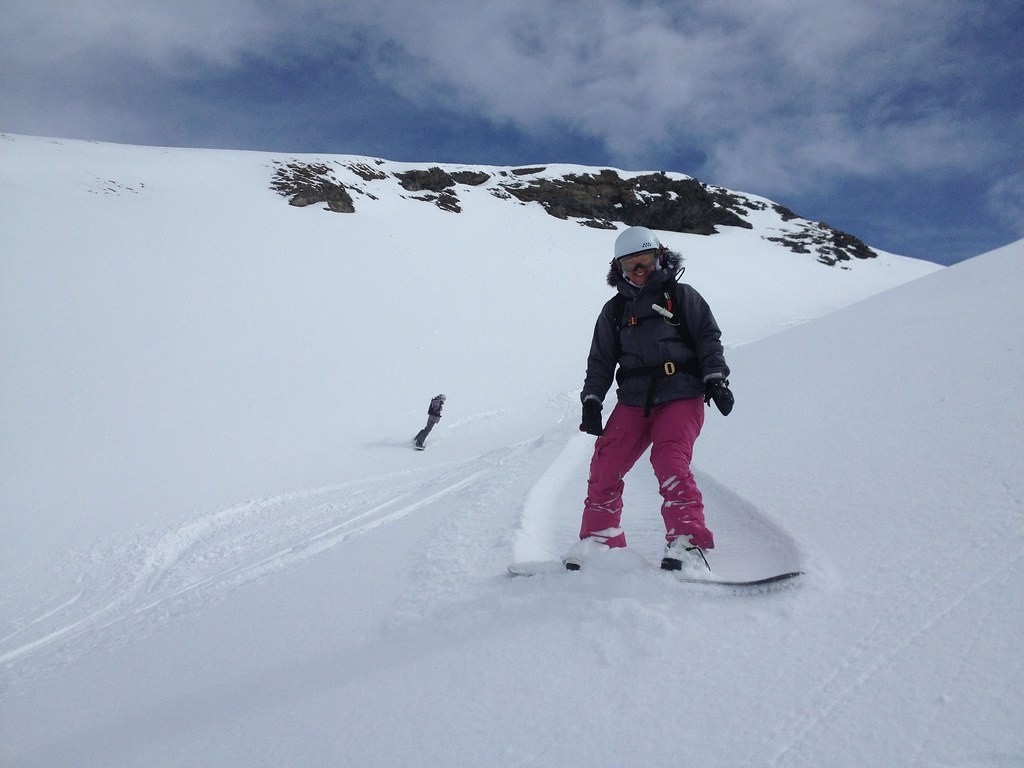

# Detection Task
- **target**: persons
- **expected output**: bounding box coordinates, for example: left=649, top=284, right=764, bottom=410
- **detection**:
left=565, top=227, right=734, bottom=581
left=413, top=393, right=447, bottom=451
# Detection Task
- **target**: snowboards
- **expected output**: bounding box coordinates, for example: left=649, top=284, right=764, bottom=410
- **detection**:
left=416, top=445, right=425, bottom=450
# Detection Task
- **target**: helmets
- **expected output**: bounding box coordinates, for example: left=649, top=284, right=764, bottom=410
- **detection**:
left=615, top=225, right=658, bottom=259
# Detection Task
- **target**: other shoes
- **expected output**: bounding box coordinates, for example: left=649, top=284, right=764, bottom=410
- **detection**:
left=660, top=544, right=712, bottom=572
left=565, top=560, right=580, bottom=569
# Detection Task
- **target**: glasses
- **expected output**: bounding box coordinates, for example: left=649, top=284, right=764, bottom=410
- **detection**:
left=619, top=249, right=656, bottom=272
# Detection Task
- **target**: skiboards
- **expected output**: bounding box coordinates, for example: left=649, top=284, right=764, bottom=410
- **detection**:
left=507, top=554, right=804, bottom=587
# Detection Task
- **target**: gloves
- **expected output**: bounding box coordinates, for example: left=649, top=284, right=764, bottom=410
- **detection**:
left=580, top=394, right=603, bottom=435
left=703, top=373, right=735, bottom=415
